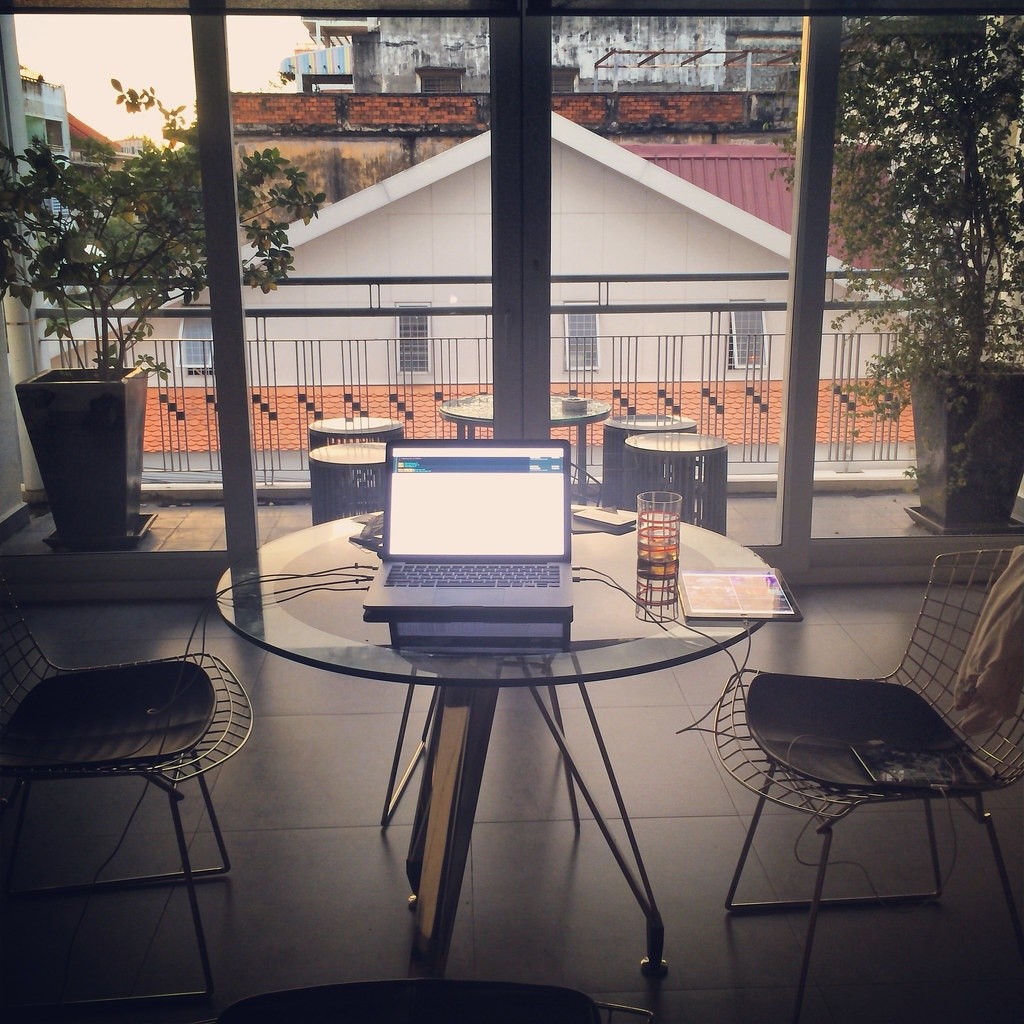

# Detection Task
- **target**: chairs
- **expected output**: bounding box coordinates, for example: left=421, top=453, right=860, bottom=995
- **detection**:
left=378, top=433, right=602, bottom=834
left=0, top=568, right=254, bottom=1024
left=711, top=542, right=1024, bottom=1024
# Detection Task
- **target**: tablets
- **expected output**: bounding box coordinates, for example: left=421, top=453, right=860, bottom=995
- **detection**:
left=678, top=567, right=803, bottom=622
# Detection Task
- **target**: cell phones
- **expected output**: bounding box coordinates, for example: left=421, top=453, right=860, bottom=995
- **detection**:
left=573, top=508, right=636, bottom=528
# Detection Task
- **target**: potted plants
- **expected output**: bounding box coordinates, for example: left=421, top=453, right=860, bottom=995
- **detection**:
left=757, top=15, right=1024, bottom=533
left=0, top=81, right=328, bottom=552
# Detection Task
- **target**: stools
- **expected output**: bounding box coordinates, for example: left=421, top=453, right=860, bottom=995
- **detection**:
left=623, top=431, right=730, bottom=537
left=601, top=414, right=697, bottom=509
left=307, top=442, right=388, bottom=527
left=309, top=418, right=404, bottom=451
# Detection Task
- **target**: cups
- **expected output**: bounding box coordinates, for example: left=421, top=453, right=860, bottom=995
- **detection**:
left=635, top=561, right=679, bottom=623
left=638, top=492, right=682, bottom=563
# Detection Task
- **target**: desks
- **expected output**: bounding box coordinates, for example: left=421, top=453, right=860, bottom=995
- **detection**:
left=439, top=392, right=611, bottom=506
left=214, top=506, right=776, bottom=979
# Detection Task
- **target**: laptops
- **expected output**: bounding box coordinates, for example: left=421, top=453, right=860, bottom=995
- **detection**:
left=362, top=439, right=574, bottom=612
